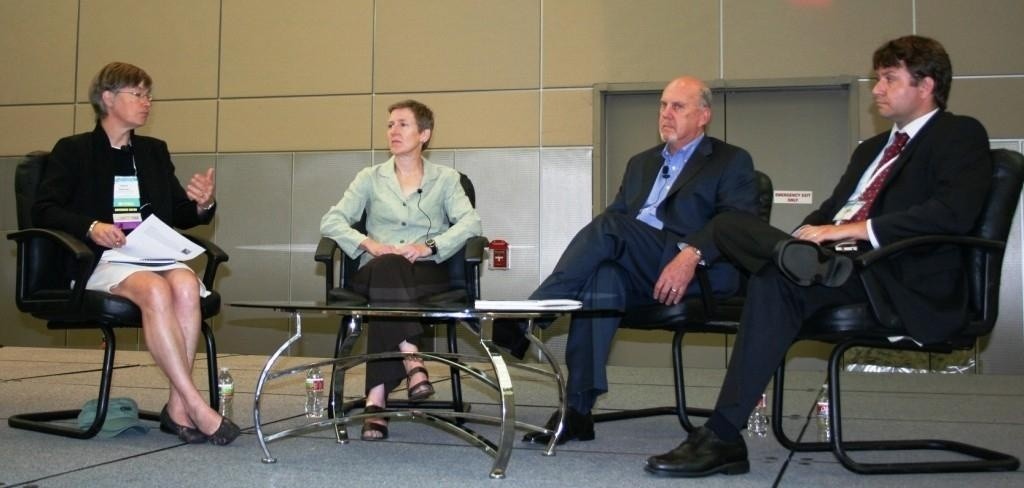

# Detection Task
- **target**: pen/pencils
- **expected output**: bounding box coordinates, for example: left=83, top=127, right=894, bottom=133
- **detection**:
left=115, top=222, right=124, bottom=247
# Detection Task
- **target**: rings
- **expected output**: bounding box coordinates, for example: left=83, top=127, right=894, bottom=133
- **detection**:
left=671, top=288, right=678, bottom=294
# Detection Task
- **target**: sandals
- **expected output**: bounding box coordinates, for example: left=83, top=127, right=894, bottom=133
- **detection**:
left=362, top=405, right=391, bottom=441
left=408, top=367, right=435, bottom=400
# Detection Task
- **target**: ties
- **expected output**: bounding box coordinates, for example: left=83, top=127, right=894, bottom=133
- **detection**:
left=838, top=132, right=909, bottom=226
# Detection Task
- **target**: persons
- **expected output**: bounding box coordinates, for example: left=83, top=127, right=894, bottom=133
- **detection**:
left=317, top=100, right=482, bottom=444
left=646, top=36, right=993, bottom=480
left=492, top=76, right=763, bottom=447
left=38, top=62, right=241, bottom=447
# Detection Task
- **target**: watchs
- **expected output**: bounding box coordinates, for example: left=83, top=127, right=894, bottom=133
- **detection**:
left=691, top=244, right=703, bottom=258
left=425, top=240, right=438, bottom=256
left=199, top=202, right=215, bottom=211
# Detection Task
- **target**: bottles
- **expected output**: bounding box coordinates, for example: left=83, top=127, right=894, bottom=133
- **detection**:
left=817, top=384, right=832, bottom=443
left=218, top=368, right=234, bottom=420
left=748, top=393, right=766, bottom=437
left=305, top=363, right=324, bottom=418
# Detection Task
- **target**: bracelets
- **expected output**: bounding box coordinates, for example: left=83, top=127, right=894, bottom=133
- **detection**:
left=87, top=219, right=101, bottom=237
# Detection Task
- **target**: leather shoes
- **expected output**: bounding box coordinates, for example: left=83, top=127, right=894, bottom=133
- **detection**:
left=160, top=404, right=207, bottom=444
left=645, top=425, right=749, bottom=477
left=778, top=239, right=854, bottom=289
left=467, top=317, right=531, bottom=359
left=189, top=408, right=240, bottom=445
left=522, top=406, right=594, bottom=444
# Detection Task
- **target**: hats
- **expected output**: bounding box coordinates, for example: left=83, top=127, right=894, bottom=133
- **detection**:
left=78, top=398, right=149, bottom=439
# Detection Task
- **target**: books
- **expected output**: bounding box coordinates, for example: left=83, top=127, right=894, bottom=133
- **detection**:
left=474, top=299, right=583, bottom=312
left=100, top=213, right=208, bottom=268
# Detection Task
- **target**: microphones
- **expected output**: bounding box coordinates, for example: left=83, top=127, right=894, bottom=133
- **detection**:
left=642, top=165, right=671, bottom=209
left=418, top=187, right=432, bottom=240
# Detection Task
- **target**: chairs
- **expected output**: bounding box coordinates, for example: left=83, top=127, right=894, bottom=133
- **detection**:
left=314, top=171, right=493, bottom=420
left=772, top=146, right=1023, bottom=474
left=6, top=149, right=228, bottom=439
left=593, top=170, right=772, bottom=432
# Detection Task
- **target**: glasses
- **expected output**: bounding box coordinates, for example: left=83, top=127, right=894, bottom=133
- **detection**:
left=113, top=90, right=152, bottom=101
